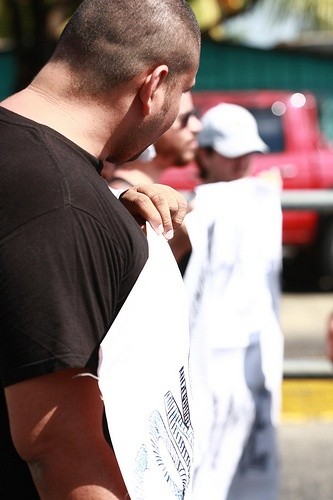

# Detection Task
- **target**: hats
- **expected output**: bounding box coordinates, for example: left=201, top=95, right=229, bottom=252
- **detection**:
left=198, top=102, right=269, bottom=158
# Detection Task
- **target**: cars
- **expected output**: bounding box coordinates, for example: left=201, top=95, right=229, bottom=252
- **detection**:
left=155, top=90, right=333, bottom=290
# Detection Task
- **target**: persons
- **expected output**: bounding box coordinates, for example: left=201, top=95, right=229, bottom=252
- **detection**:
left=181, top=103, right=267, bottom=207
left=108, top=81, right=202, bottom=189
left=0, top=0, right=201, bottom=499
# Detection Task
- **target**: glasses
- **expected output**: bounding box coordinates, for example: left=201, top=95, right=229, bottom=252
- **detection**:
left=178, top=106, right=200, bottom=129
